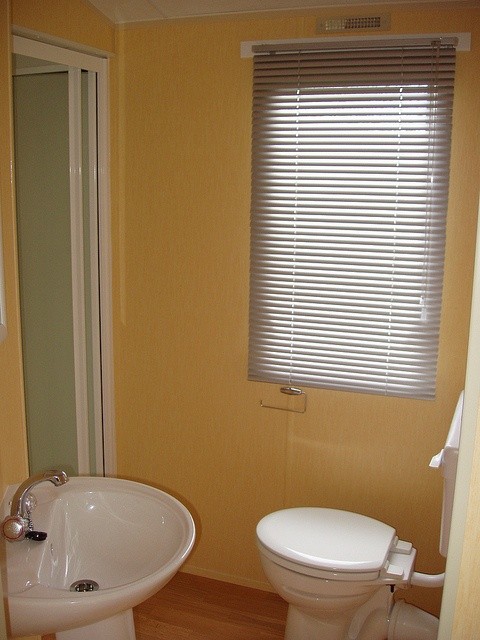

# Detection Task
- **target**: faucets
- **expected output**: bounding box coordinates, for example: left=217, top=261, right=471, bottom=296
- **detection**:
left=1, top=469, right=70, bottom=542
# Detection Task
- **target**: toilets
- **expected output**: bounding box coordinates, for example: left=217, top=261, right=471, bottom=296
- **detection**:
left=255, top=390, right=464, bottom=640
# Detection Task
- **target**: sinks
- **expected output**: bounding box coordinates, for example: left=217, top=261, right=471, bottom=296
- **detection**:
left=37, top=488, right=185, bottom=594
